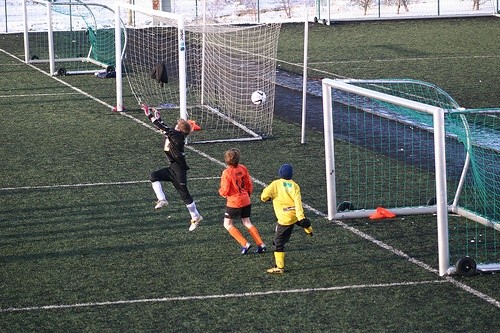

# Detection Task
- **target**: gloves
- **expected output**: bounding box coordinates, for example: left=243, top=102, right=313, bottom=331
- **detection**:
left=152, top=107, right=161, bottom=120
left=141, top=104, right=152, bottom=119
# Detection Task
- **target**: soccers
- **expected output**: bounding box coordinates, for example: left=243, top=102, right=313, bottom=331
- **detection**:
left=251, top=90, right=267, bottom=106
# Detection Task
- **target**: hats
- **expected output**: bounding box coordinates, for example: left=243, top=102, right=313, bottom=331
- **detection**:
left=279, top=164, right=294, bottom=180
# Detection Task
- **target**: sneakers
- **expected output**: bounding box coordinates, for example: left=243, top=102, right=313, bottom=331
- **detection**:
left=267, top=266, right=285, bottom=274
left=188, top=215, right=203, bottom=231
left=254, top=245, right=266, bottom=253
left=240, top=242, right=251, bottom=256
left=155, top=199, right=168, bottom=209
left=308, top=232, right=313, bottom=236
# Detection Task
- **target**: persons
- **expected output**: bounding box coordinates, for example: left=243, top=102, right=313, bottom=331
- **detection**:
left=218, top=148, right=266, bottom=255
left=139, top=101, right=203, bottom=231
left=260, top=163, right=313, bottom=273
